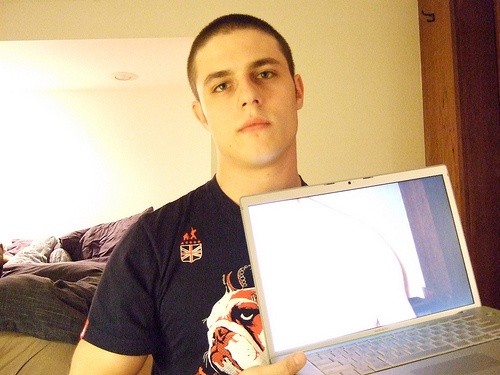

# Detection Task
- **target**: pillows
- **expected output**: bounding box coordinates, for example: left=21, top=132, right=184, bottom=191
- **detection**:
left=3, top=235, right=57, bottom=270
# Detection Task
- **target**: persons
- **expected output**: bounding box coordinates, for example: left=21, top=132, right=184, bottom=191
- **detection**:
left=68, top=13, right=310, bottom=375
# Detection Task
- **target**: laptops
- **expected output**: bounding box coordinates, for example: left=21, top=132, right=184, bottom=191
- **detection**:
left=239, top=164, right=500, bottom=375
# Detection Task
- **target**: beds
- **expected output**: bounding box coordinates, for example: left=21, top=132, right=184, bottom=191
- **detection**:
left=0, top=206, right=154, bottom=375
